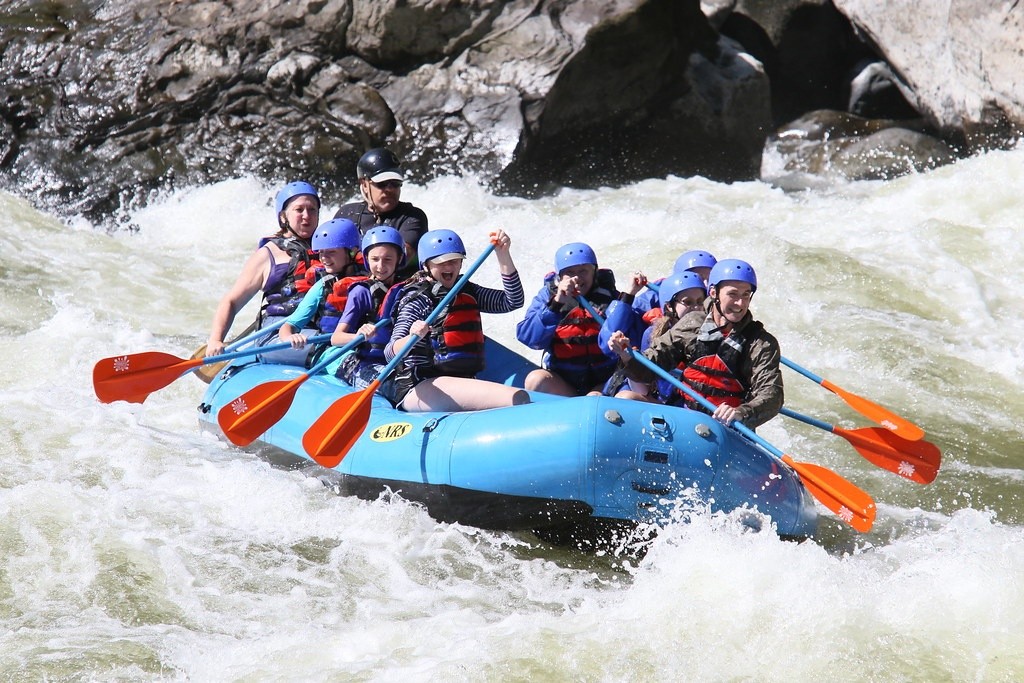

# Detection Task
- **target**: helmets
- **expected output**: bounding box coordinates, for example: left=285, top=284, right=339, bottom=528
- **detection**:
left=311, top=216, right=361, bottom=254
left=555, top=243, right=598, bottom=274
left=276, top=181, right=321, bottom=224
left=357, top=148, right=399, bottom=181
left=675, top=251, right=716, bottom=275
left=659, top=272, right=708, bottom=315
left=417, top=229, right=467, bottom=270
left=361, top=226, right=407, bottom=272
left=706, top=259, right=757, bottom=299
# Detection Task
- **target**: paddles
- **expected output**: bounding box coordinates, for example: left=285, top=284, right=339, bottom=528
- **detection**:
left=190, top=321, right=258, bottom=385
left=636, top=278, right=926, bottom=443
left=780, top=407, right=941, bottom=485
left=572, top=289, right=605, bottom=326
left=218, top=318, right=391, bottom=446
left=620, top=333, right=877, bottom=533
left=301, top=232, right=498, bottom=468
left=97, top=314, right=292, bottom=404
left=94, top=333, right=332, bottom=401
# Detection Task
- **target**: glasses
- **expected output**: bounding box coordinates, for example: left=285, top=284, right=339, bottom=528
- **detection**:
left=374, top=181, right=402, bottom=190
left=674, top=295, right=704, bottom=307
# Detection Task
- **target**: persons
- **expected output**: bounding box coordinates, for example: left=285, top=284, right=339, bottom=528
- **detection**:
left=598, top=250, right=785, bottom=434
left=516, top=242, right=623, bottom=396
left=206, top=147, right=530, bottom=411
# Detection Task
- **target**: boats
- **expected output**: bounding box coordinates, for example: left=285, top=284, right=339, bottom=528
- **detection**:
left=197, top=344, right=815, bottom=554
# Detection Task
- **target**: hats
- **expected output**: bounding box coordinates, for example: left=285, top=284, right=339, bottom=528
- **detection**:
left=369, top=171, right=405, bottom=185
left=430, top=253, right=468, bottom=265
